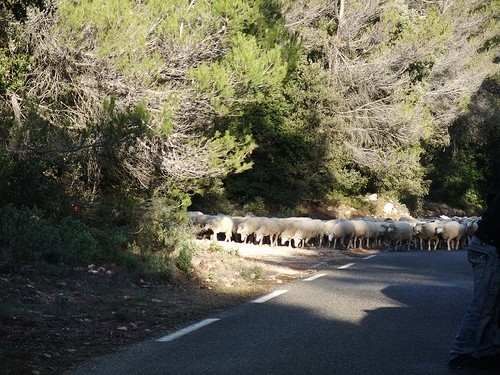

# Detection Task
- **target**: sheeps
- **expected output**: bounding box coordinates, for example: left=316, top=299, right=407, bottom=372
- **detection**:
left=186, top=203, right=483, bottom=252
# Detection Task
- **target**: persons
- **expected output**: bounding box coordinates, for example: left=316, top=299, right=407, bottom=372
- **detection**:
left=447, top=183, right=500, bottom=370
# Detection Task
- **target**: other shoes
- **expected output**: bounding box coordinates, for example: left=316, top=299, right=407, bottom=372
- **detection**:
left=444, top=353, right=500, bottom=375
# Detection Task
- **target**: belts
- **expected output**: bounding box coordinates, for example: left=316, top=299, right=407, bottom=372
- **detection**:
left=474, top=229, right=500, bottom=248
